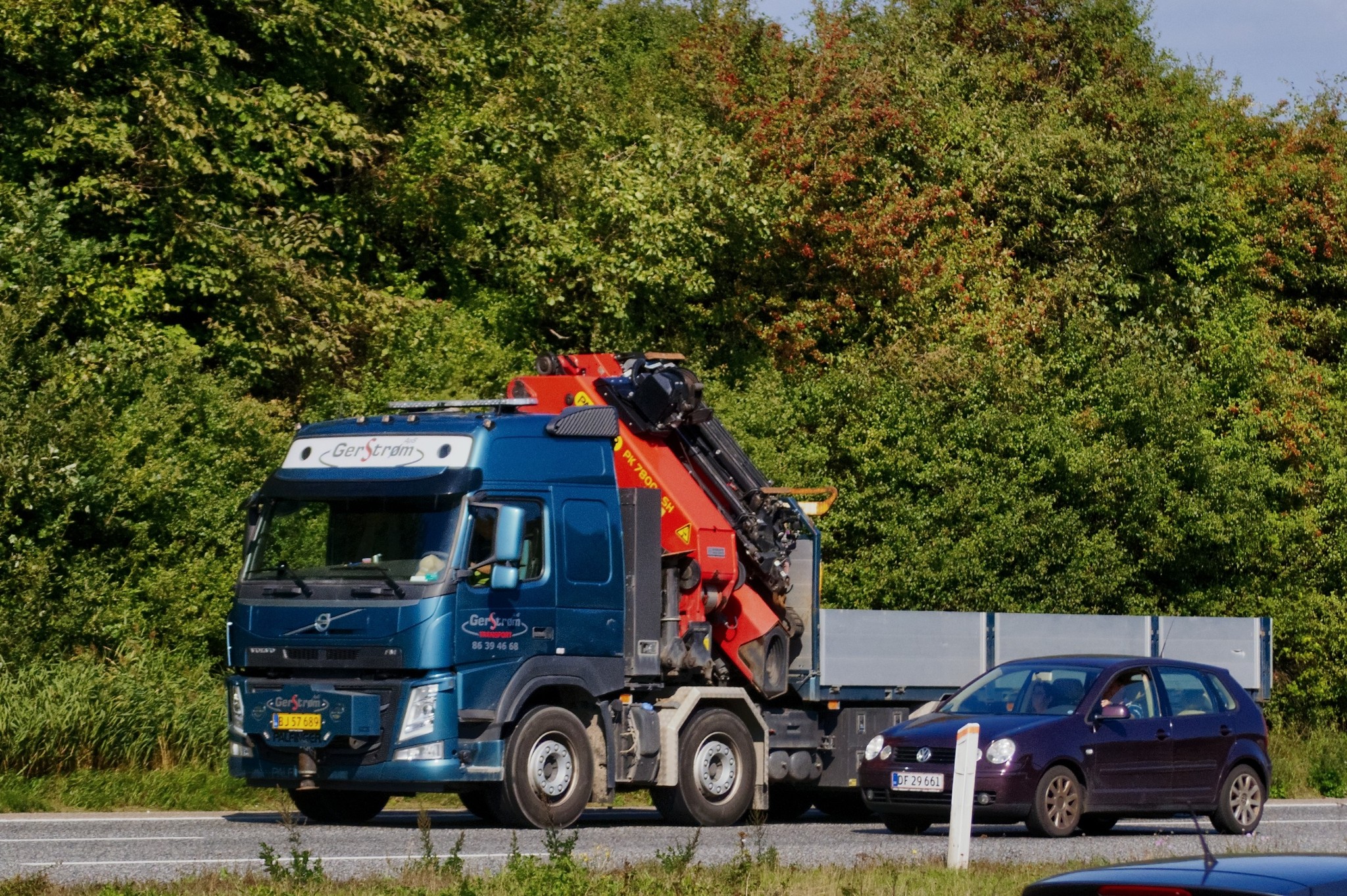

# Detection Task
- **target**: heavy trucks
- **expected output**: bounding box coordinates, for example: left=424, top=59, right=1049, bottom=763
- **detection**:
left=224, top=353, right=1272, bottom=829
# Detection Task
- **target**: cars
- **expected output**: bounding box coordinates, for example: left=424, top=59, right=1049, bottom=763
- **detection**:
left=857, top=654, right=1273, bottom=838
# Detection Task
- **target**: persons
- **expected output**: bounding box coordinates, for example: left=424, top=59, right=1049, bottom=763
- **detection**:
left=1099, top=672, right=1143, bottom=719
left=1033, top=682, right=1054, bottom=714
left=420, top=515, right=494, bottom=587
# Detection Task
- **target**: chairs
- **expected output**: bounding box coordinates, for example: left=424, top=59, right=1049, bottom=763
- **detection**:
left=1049, top=678, right=1083, bottom=707
